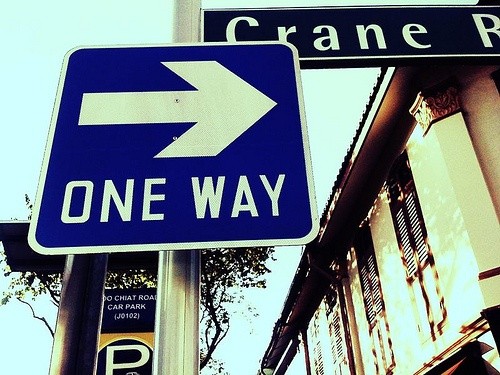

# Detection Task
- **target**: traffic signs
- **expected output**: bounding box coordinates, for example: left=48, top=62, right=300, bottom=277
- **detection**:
left=27, top=40, right=321, bottom=252
left=92, top=284, right=157, bottom=375
left=200, top=4, right=500, bottom=61
